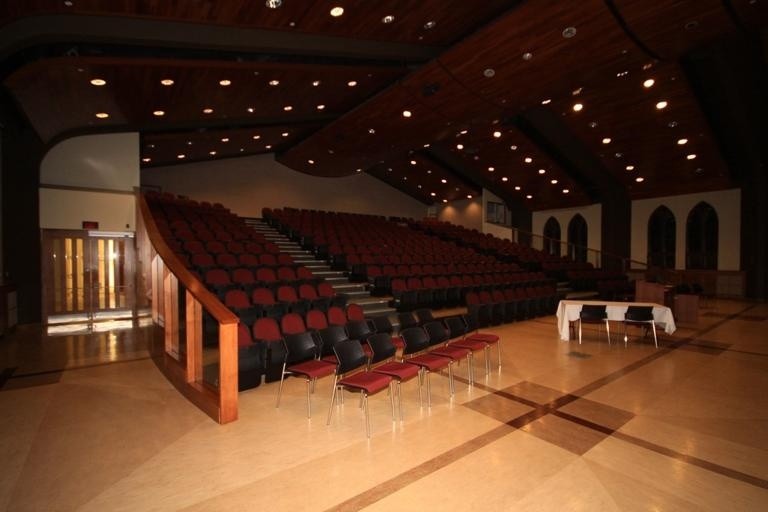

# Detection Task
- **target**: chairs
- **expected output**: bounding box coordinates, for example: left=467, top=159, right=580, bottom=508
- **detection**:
left=140, top=189, right=501, bottom=438
left=621, top=305, right=657, bottom=349
left=501, top=236, right=629, bottom=324
left=575, top=304, right=610, bottom=348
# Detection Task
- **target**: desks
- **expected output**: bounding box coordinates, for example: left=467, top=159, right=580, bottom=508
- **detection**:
left=559, top=299, right=669, bottom=341
